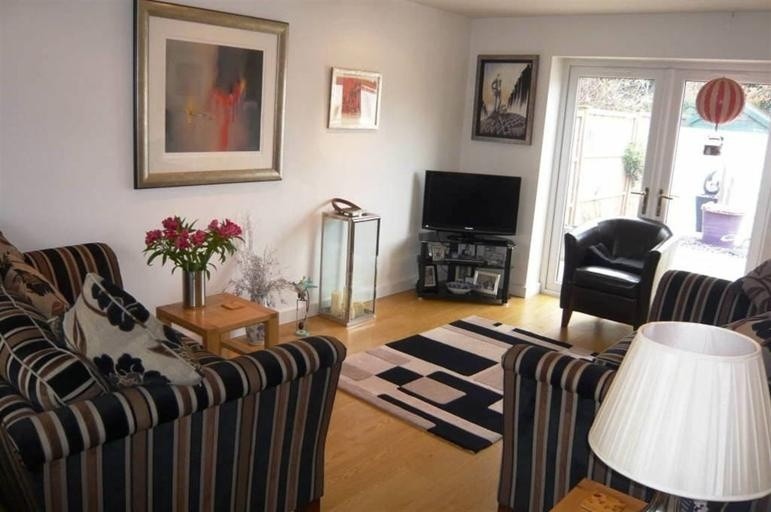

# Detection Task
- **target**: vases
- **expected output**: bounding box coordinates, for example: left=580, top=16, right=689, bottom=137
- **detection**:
left=183, top=271, right=205, bottom=310
left=246, top=294, right=266, bottom=345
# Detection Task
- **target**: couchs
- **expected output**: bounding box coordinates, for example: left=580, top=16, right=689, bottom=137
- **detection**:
left=497, top=259, right=771, bottom=512
left=1, top=243, right=347, bottom=512
left=560, top=217, right=673, bottom=332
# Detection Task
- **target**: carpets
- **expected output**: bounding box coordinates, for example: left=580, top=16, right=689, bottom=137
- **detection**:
left=337, top=316, right=599, bottom=454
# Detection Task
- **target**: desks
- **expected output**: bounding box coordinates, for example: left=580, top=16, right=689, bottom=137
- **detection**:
left=417, top=231, right=516, bottom=304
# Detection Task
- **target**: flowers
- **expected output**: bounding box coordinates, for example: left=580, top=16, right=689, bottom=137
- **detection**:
left=142, top=215, right=246, bottom=282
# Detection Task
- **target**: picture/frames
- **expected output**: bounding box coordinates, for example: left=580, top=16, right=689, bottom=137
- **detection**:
left=132, top=0, right=289, bottom=189
left=471, top=54, right=539, bottom=146
left=432, top=245, right=444, bottom=262
left=425, top=264, right=439, bottom=294
left=328, top=67, right=383, bottom=130
left=473, top=271, right=501, bottom=295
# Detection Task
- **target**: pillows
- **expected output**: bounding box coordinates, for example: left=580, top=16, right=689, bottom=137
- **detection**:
left=723, top=311, right=771, bottom=381
left=45, top=272, right=206, bottom=392
left=741, top=259, right=771, bottom=308
left=1, top=228, right=71, bottom=318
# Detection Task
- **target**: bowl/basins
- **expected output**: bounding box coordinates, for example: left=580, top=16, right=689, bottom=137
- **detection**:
left=446, top=282, right=473, bottom=295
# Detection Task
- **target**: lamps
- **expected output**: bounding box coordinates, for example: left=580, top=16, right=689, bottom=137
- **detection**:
left=588, top=322, right=771, bottom=512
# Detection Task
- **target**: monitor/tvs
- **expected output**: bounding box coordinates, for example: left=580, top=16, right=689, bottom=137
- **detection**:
left=421, top=169, right=522, bottom=244
